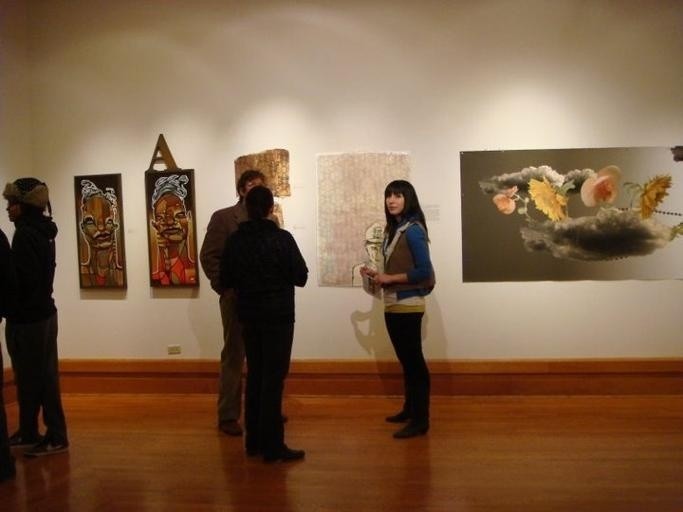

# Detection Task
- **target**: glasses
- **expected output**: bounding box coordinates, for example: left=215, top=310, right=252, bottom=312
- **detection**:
left=246, top=181, right=264, bottom=187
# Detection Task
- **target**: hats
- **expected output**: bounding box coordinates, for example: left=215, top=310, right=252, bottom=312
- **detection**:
left=2, top=178, right=49, bottom=211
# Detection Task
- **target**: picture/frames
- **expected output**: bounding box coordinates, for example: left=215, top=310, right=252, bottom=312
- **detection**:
left=73, top=172, right=128, bottom=291
left=144, top=168, right=200, bottom=289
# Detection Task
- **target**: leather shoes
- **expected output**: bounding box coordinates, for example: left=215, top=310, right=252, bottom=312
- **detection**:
left=385, top=407, right=412, bottom=422
left=247, top=443, right=286, bottom=456
left=219, top=420, right=244, bottom=437
left=393, top=419, right=429, bottom=438
left=260, top=446, right=303, bottom=461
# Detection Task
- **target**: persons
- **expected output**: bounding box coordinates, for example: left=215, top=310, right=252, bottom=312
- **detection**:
left=199, top=170, right=287, bottom=435
left=217, top=185, right=308, bottom=462
left=0, top=228, right=18, bottom=483
left=3, top=177, right=69, bottom=457
left=359, top=180, right=436, bottom=439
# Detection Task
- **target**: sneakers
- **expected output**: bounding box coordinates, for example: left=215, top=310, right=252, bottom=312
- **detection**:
left=25, top=437, right=70, bottom=457
left=8, top=430, right=41, bottom=448
